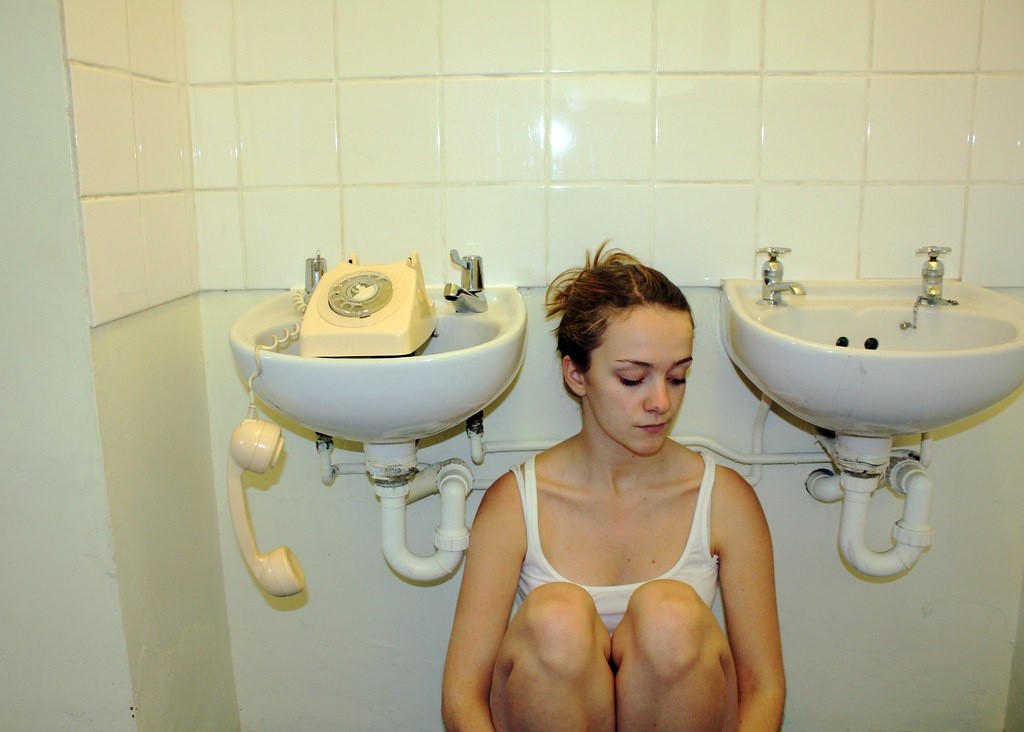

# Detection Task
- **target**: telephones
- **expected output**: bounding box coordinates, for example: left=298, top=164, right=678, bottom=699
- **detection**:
left=227, top=406, right=306, bottom=598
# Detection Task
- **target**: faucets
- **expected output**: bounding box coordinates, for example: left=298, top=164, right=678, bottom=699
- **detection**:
left=754, top=246, right=807, bottom=308
left=443, top=247, right=488, bottom=313
left=304, top=249, right=327, bottom=303
left=914, top=244, right=954, bottom=305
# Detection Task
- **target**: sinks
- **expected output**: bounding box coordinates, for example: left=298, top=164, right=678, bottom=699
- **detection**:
left=233, top=312, right=527, bottom=446
left=741, top=299, right=1023, bottom=437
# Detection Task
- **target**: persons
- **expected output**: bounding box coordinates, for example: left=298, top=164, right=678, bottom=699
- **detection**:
left=437, top=240, right=787, bottom=732
